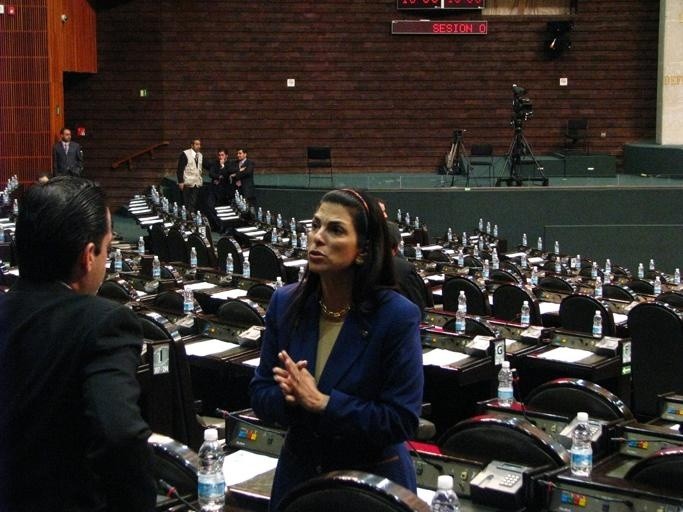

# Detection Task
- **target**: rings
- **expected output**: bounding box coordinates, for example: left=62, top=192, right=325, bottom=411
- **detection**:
left=295, top=376, right=300, bottom=383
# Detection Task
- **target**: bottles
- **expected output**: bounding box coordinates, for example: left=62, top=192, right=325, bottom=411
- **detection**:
left=493, top=225, right=498, bottom=238
left=276, top=214, right=283, bottom=229
left=482, top=259, right=490, bottom=280
left=171, top=201, right=178, bottom=216
left=594, top=277, right=602, bottom=300
left=414, top=217, right=420, bottom=229
left=447, top=228, right=453, bottom=242
left=289, top=217, right=297, bottom=233
left=455, top=306, right=466, bottom=336
left=266, top=211, right=272, bottom=225
left=462, top=232, right=467, bottom=246
left=151, top=185, right=159, bottom=204
left=530, top=266, right=537, bottom=286
left=12, top=199, right=19, bottom=215
left=151, top=256, right=161, bottom=280
left=415, top=243, right=422, bottom=261
left=554, top=257, right=562, bottom=275
left=196, top=211, right=202, bottom=225
left=274, top=277, right=284, bottom=289
left=242, top=257, right=251, bottom=277
left=3, top=174, right=20, bottom=193
left=225, top=252, right=234, bottom=274
left=0, top=225, right=5, bottom=245
left=575, top=255, right=582, bottom=272
left=674, top=268, right=681, bottom=284
left=196, top=428, right=227, bottom=510
left=300, top=233, right=307, bottom=250
left=591, top=310, right=603, bottom=339
left=161, top=197, right=169, bottom=212
left=605, top=259, right=612, bottom=272
left=458, top=250, right=464, bottom=268
left=591, top=261, right=598, bottom=280
left=114, top=249, right=123, bottom=273
left=653, top=276, right=662, bottom=294
left=404, top=212, right=410, bottom=225
left=520, top=300, right=532, bottom=328
left=2, top=187, right=9, bottom=204
left=400, top=240, right=405, bottom=258
left=183, top=285, right=193, bottom=314
left=479, top=236, right=484, bottom=251
left=473, top=244, right=478, bottom=259
left=491, top=248, right=498, bottom=264
left=297, top=267, right=307, bottom=283
left=291, top=231, right=297, bottom=248
left=181, top=204, right=187, bottom=220
left=487, top=222, right=490, bottom=235
left=138, top=236, right=146, bottom=256
left=458, top=291, right=467, bottom=313
left=497, top=360, right=515, bottom=409
left=257, top=207, right=263, bottom=222
left=553, top=240, right=560, bottom=255
left=271, top=228, right=278, bottom=244
left=604, top=272, right=610, bottom=285
left=521, top=252, right=527, bottom=270
left=637, top=262, right=645, bottom=279
left=431, top=475, right=462, bottom=511
left=234, top=189, right=246, bottom=212
left=522, top=233, right=528, bottom=247
left=397, top=208, right=402, bottom=224
left=568, top=412, right=595, bottom=479
left=537, top=237, right=543, bottom=251
left=648, top=258, right=655, bottom=271
left=478, top=218, right=484, bottom=232
left=190, top=247, right=198, bottom=268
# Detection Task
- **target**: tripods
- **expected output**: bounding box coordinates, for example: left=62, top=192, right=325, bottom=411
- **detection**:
left=495, top=113, right=549, bottom=187
left=441, top=129, right=479, bottom=187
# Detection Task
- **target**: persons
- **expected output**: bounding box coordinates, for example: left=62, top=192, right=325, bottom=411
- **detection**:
left=223, top=145, right=255, bottom=207
left=176, top=137, right=212, bottom=214
left=207, top=146, right=232, bottom=209
left=384, top=221, right=431, bottom=323
left=370, top=197, right=389, bottom=221
left=52, top=126, right=84, bottom=179
left=250, top=188, right=428, bottom=511
left=0, top=176, right=160, bottom=511
left=34, top=171, right=49, bottom=183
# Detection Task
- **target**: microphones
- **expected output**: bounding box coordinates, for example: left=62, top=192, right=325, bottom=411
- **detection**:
left=537, top=479, right=634, bottom=507
left=130, top=295, right=152, bottom=310
left=158, top=479, right=202, bottom=512
left=610, top=437, right=683, bottom=446
left=510, top=368, right=537, bottom=425
left=191, top=310, right=214, bottom=327
left=216, top=408, right=286, bottom=437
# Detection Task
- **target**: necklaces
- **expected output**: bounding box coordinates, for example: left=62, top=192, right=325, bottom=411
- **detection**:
left=317, top=296, right=354, bottom=323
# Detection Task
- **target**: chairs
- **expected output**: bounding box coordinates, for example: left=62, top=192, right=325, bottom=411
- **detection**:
left=465, top=145, right=497, bottom=187
left=305, top=143, right=336, bottom=187
